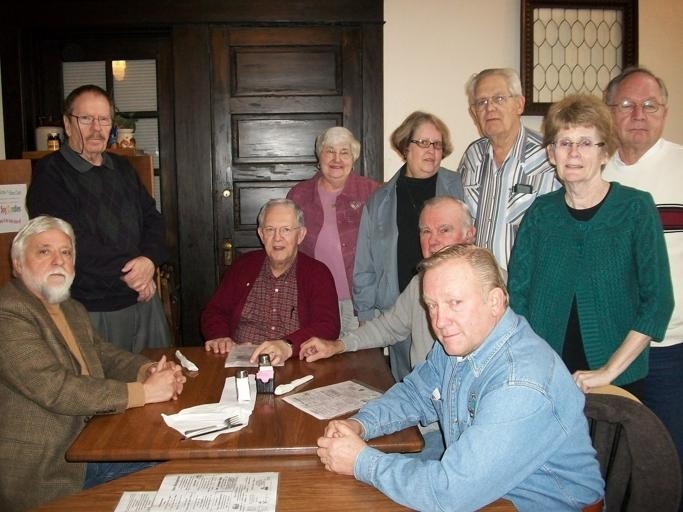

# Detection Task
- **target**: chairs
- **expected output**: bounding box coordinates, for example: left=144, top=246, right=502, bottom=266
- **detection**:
left=583, top=385, right=683, bottom=512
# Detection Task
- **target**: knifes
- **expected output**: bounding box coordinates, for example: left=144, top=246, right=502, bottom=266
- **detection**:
left=187, top=423, right=245, bottom=440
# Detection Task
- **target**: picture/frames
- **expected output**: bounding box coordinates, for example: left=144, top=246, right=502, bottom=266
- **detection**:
left=520, top=0, right=639, bottom=116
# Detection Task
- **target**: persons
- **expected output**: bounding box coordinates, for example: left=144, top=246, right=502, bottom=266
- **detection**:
left=286, top=126, right=382, bottom=339
left=507, top=93, right=674, bottom=403
left=25, top=85, right=173, bottom=354
left=317, top=243, right=606, bottom=512
left=0, top=214, right=186, bottom=508
left=458, top=68, right=564, bottom=286
left=600, top=67, right=683, bottom=468
left=299, top=196, right=477, bottom=371
left=351, top=112, right=463, bottom=384
left=200, top=198, right=341, bottom=367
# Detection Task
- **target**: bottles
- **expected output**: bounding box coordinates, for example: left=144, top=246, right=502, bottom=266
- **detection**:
left=46, top=131, right=59, bottom=151
left=233, top=369, right=251, bottom=401
left=109, top=129, right=117, bottom=150
left=256, top=353, right=274, bottom=393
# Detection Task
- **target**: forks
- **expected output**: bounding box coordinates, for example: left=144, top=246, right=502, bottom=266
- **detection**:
left=183, top=413, right=243, bottom=435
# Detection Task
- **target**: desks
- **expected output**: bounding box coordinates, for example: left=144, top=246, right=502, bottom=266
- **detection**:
left=22, top=461, right=517, bottom=512
left=64, top=347, right=424, bottom=463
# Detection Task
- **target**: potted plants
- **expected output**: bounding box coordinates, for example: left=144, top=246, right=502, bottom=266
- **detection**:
left=113, top=115, right=136, bottom=148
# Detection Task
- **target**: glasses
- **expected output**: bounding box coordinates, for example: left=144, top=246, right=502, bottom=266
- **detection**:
left=607, top=100, right=664, bottom=113
left=472, top=93, right=514, bottom=111
left=263, top=226, right=300, bottom=234
left=412, top=138, right=444, bottom=149
left=67, top=114, right=111, bottom=126
left=551, top=138, right=606, bottom=150
